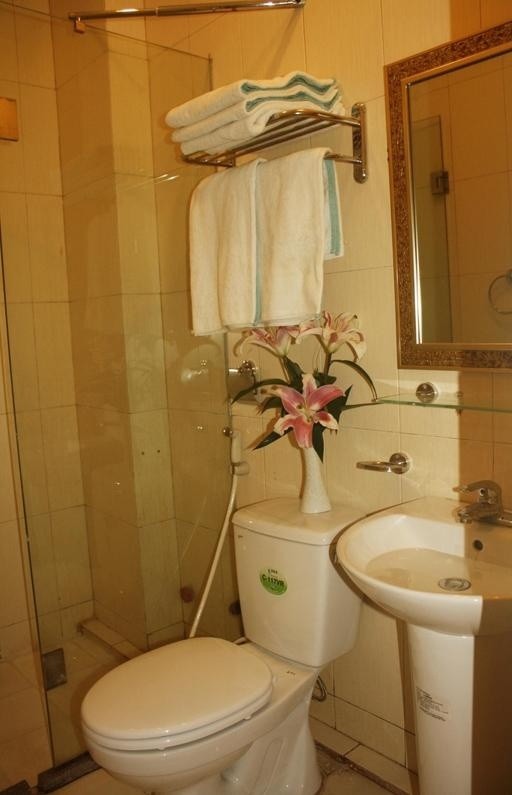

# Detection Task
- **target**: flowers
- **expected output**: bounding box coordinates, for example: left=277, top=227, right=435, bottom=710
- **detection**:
left=225, top=312, right=379, bottom=462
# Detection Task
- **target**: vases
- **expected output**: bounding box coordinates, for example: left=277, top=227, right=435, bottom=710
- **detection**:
left=301, top=441, right=333, bottom=514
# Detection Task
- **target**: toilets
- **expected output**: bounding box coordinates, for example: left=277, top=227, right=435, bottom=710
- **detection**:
left=78, top=495, right=363, bottom=795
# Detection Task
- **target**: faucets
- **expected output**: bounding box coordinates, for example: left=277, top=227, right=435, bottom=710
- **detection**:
left=455, top=479, right=504, bottom=524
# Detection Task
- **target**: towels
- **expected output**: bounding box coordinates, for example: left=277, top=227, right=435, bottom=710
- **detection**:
left=189, top=146, right=343, bottom=334
left=164, top=67, right=348, bottom=160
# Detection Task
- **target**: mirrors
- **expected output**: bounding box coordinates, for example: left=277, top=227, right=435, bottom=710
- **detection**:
left=384, top=22, right=512, bottom=370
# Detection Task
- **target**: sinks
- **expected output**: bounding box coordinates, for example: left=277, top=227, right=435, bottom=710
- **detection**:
left=336, top=495, right=512, bottom=637
